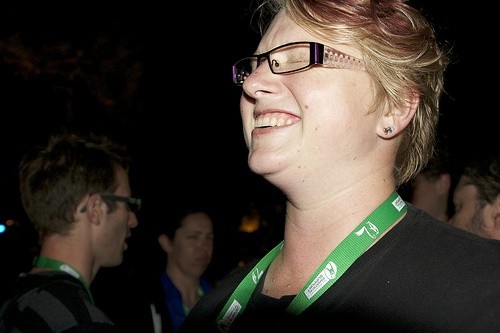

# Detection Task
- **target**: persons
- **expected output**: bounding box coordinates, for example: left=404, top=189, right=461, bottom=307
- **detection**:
left=176, top=0, right=500, bottom=333
left=396, top=141, right=450, bottom=224
left=0, top=131, right=137, bottom=333
left=112, top=201, right=213, bottom=333
left=445, top=146, right=500, bottom=242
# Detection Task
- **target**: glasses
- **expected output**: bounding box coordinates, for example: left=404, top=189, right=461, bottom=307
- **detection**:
left=232, top=41, right=371, bottom=86
left=79, top=195, right=142, bottom=213
